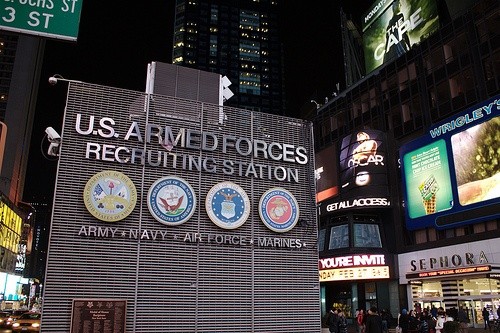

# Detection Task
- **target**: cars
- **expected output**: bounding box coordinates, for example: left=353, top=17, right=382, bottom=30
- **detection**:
left=0, top=305, right=41, bottom=333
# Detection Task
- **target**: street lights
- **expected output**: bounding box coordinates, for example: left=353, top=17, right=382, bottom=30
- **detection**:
left=39, top=283, right=43, bottom=299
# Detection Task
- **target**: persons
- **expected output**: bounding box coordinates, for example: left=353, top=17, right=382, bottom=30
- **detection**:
left=497, top=305, right=500, bottom=319
left=383, top=0, right=412, bottom=64
left=352, top=131, right=378, bottom=164
left=328, top=301, right=472, bottom=333
left=482, top=307, right=491, bottom=329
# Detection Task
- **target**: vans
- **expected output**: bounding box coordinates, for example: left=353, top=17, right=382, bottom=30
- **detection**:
left=1, top=300, right=20, bottom=311
